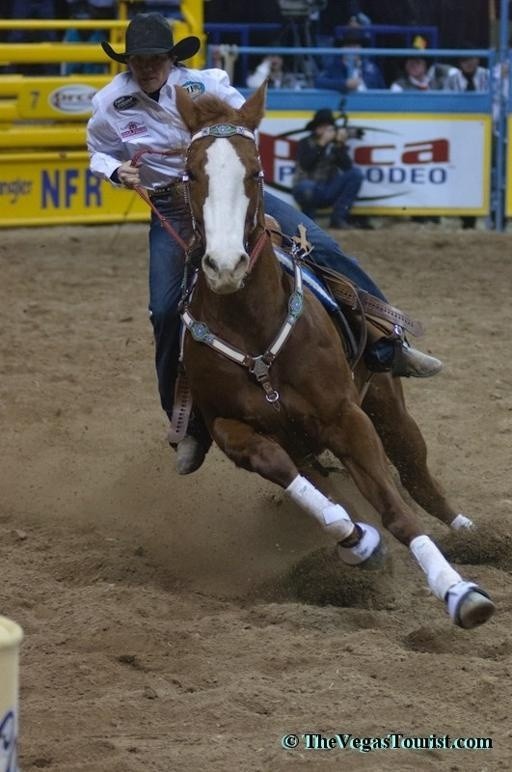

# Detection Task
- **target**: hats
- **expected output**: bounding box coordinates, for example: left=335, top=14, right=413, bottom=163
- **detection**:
left=305, top=110, right=336, bottom=130
left=101, top=11, right=201, bottom=64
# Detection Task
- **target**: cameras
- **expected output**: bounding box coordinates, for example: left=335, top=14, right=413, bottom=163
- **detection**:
left=332, top=124, right=365, bottom=142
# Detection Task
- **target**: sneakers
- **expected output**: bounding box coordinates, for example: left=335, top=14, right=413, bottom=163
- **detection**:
left=176, top=433, right=206, bottom=475
left=364, top=336, right=442, bottom=378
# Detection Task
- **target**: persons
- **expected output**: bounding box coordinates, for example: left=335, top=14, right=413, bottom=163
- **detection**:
left=86, top=11, right=443, bottom=475
left=0, top=0, right=512, bottom=229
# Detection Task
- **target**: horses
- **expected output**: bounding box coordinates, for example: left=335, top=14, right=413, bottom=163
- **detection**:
left=173, top=78, right=496, bottom=633
